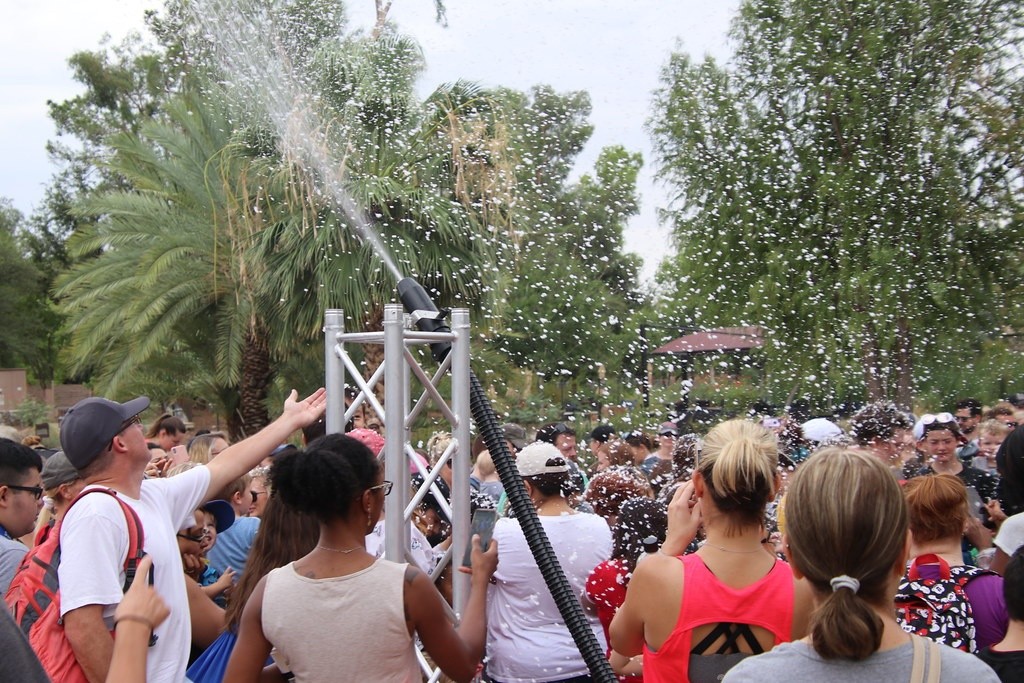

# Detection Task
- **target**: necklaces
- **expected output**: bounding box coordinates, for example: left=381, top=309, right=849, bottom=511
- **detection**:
left=318, top=545, right=363, bottom=554
left=704, top=541, right=763, bottom=553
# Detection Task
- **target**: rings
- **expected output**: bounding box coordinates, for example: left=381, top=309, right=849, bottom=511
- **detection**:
left=187, top=570, right=195, bottom=574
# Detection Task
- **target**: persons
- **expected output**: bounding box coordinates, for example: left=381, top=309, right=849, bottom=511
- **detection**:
left=223, top=432, right=500, bottom=683
left=766, top=392, right=1024, bottom=682
left=0, top=412, right=320, bottom=683
left=59, top=388, right=326, bottom=683
left=720, top=447, right=1005, bottom=682
left=608, top=418, right=820, bottom=683
left=298, top=384, right=707, bottom=683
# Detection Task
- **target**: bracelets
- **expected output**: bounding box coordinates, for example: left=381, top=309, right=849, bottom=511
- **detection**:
left=113, top=615, right=155, bottom=634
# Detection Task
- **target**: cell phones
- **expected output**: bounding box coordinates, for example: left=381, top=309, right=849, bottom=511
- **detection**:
left=171, top=445, right=188, bottom=467
left=461, top=509, right=498, bottom=566
left=965, top=486, right=990, bottom=525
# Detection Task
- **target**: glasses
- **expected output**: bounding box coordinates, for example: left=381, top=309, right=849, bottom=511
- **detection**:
left=356, top=481, right=393, bottom=502
left=660, top=432, right=676, bottom=437
left=7, top=484, right=43, bottom=500
left=446, top=458, right=452, bottom=468
left=108, top=413, right=142, bottom=451
left=1003, top=422, right=1018, bottom=428
left=251, top=491, right=267, bottom=501
left=954, top=416, right=974, bottom=422
left=177, top=528, right=209, bottom=543
left=550, top=424, right=571, bottom=436
left=921, top=411, right=960, bottom=427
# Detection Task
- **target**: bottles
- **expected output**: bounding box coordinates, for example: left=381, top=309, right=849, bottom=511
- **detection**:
left=34, top=499, right=54, bottom=540
left=636, top=537, right=664, bottom=568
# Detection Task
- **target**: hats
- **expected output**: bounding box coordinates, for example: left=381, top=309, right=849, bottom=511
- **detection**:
left=40, top=451, right=80, bottom=491
left=268, top=443, right=298, bottom=456
left=500, top=423, right=530, bottom=449
left=410, top=452, right=430, bottom=473
left=201, top=500, right=235, bottom=533
left=59, top=396, right=151, bottom=470
left=911, top=420, right=924, bottom=443
left=924, top=419, right=968, bottom=444
left=345, top=428, right=385, bottom=457
left=515, top=441, right=572, bottom=476
left=658, top=421, right=679, bottom=436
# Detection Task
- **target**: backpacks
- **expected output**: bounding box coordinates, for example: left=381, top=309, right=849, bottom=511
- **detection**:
left=894, top=553, right=1003, bottom=654
left=4, top=489, right=144, bottom=683
